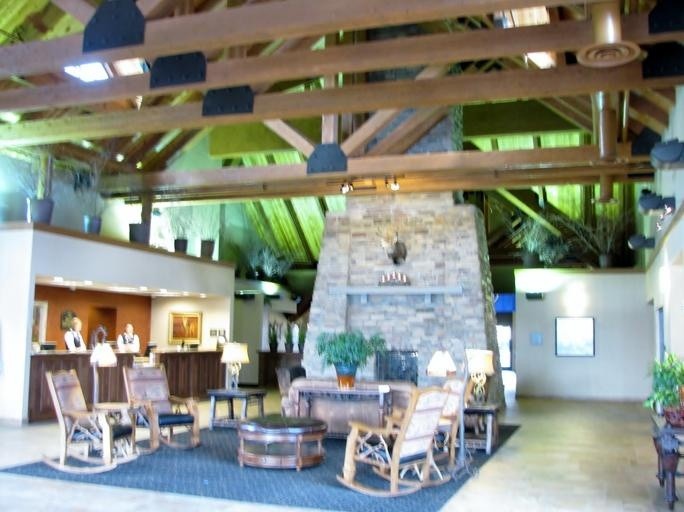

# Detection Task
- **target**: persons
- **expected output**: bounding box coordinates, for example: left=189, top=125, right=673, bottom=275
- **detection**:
left=63, top=317, right=86, bottom=352
left=117, top=322, right=140, bottom=353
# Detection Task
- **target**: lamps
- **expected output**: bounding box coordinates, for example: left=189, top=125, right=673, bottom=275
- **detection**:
left=426, top=350, right=456, bottom=380
left=465, top=349, right=496, bottom=405
left=89, top=342, right=118, bottom=412
left=220, top=343, right=250, bottom=391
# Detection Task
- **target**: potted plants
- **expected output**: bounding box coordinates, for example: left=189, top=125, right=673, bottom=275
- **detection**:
left=642, top=353, right=684, bottom=428
left=268, top=319, right=307, bottom=353
left=316, top=331, right=387, bottom=390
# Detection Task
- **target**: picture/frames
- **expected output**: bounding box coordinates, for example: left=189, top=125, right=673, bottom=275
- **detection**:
left=33, top=300, right=48, bottom=351
left=168, top=312, right=202, bottom=345
left=555, top=317, right=595, bottom=357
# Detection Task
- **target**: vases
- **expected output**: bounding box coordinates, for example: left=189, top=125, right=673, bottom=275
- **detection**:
left=26, top=198, right=54, bottom=225
left=175, top=240, right=187, bottom=254
left=83, top=215, right=101, bottom=235
left=129, top=224, right=147, bottom=244
left=201, top=241, right=214, bottom=260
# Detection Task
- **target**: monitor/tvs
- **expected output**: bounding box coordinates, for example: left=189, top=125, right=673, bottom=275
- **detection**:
left=40, top=343, right=56, bottom=350
left=144, top=344, right=157, bottom=356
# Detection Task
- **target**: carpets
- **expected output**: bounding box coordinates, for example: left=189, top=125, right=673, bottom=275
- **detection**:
left=0, top=414, right=521, bottom=512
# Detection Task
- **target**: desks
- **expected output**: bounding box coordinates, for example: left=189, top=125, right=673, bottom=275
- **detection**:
left=298, top=387, right=392, bottom=445
left=207, top=389, right=267, bottom=432
left=237, top=415, right=328, bottom=472
left=443, top=403, right=499, bottom=456
left=650, top=414, right=684, bottom=511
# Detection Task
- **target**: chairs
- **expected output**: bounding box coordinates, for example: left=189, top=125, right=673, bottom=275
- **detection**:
left=122, top=364, right=201, bottom=455
left=41, top=368, right=140, bottom=474
left=336, top=377, right=475, bottom=498
